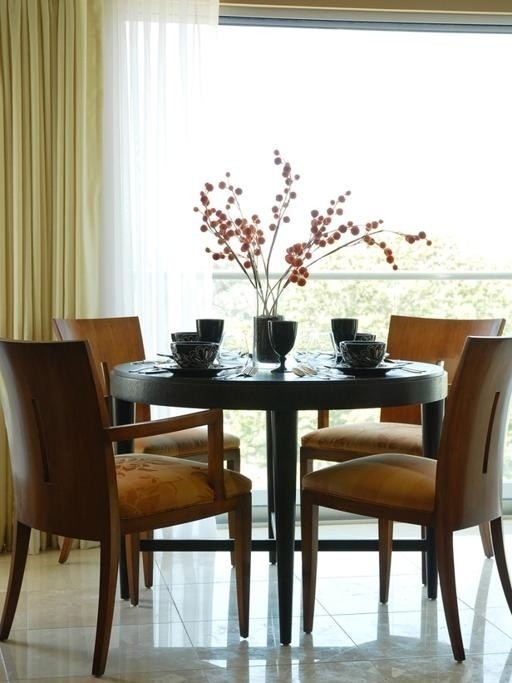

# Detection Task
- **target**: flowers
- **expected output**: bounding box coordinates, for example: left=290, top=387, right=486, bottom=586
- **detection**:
left=190, top=148, right=433, bottom=317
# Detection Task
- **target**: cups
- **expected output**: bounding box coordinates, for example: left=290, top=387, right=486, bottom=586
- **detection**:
left=196, top=319, right=224, bottom=344
left=331, top=318, right=358, bottom=363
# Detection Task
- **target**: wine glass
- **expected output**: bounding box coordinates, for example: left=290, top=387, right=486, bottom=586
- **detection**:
left=268, top=320, right=298, bottom=373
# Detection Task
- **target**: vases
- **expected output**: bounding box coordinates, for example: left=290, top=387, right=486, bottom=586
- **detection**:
left=251, top=287, right=288, bottom=368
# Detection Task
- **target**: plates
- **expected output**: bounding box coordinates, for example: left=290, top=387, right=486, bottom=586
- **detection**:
left=324, top=363, right=403, bottom=377
left=161, top=367, right=235, bottom=375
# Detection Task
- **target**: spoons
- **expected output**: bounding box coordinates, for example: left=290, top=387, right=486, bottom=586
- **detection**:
left=293, top=366, right=350, bottom=380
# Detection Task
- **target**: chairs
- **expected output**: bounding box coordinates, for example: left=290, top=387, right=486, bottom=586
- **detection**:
left=300, top=315, right=506, bottom=604
left=52, top=316, right=240, bottom=588
left=0, top=339, right=250, bottom=677
left=301, top=335, right=512, bottom=663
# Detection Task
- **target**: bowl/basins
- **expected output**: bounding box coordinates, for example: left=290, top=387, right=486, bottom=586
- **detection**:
left=169, top=332, right=219, bottom=369
left=339, top=333, right=385, bottom=368
